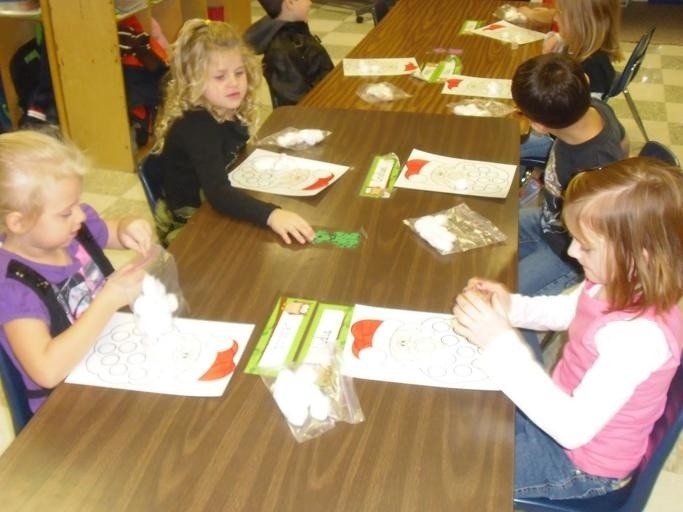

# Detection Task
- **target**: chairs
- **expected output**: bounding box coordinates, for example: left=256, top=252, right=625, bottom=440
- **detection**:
left=638, top=141, right=680, bottom=166
left=603, top=27, right=657, bottom=142
left=513, top=360, right=683, bottom=512
left=138, top=154, right=161, bottom=206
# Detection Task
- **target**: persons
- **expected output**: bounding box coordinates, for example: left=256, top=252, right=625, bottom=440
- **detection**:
left=520, top=0, right=625, bottom=167
left=243, top=0, right=334, bottom=107
left=450, top=156, right=683, bottom=501
left=511, top=52, right=629, bottom=363
left=0, top=130, right=154, bottom=415
left=148, top=18, right=315, bottom=245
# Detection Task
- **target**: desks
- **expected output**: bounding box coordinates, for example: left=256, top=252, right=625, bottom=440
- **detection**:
left=0, top=105, right=520, bottom=512
left=297, top=0, right=544, bottom=142
